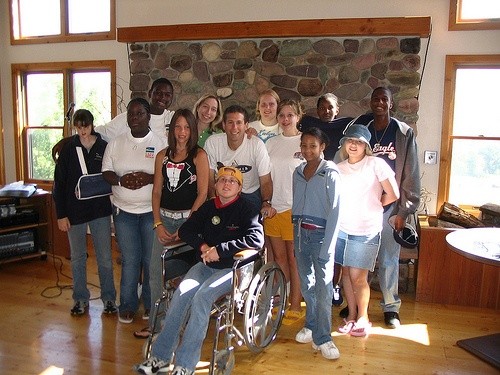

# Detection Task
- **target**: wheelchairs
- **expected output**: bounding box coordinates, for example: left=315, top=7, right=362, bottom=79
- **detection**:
left=135, top=210, right=287, bottom=375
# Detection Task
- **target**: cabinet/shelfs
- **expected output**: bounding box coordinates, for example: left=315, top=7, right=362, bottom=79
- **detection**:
left=0, top=189, right=52, bottom=263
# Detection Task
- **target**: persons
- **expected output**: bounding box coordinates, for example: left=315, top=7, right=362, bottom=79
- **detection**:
left=300, top=92, right=355, bottom=162
left=136, top=167, right=265, bottom=375
left=290, top=128, right=343, bottom=360
left=333, top=86, right=420, bottom=328
left=336, top=123, right=400, bottom=337
left=263, top=99, right=324, bottom=327
left=102, top=97, right=168, bottom=324
left=52, top=78, right=284, bottom=309
left=51, top=108, right=118, bottom=316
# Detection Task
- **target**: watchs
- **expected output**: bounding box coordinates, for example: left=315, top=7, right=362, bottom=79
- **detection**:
left=262, top=200, right=271, bottom=207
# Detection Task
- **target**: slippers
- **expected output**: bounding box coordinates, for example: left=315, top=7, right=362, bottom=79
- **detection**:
left=133, top=327, right=151, bottom=339
left=282, top=307, right=307, bottom=325
left=339, top=318, right=356, bottom=333
left=272, top=301, right=289, bottom=321
left=350, top=321, right=372, bottom=337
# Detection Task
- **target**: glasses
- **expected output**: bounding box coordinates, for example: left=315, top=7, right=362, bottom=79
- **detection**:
left=218, top=178, right=241, bottom=186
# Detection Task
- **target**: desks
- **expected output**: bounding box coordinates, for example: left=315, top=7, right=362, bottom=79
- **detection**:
left=445, top=227, right=500, bottom=370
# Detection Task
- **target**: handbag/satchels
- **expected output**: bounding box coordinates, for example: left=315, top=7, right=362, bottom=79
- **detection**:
left=75, top=173, right=113, bottom=200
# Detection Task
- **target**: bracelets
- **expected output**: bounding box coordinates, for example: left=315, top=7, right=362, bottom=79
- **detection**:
left=152, top=222, right=163, bottom=230
left=118, top=176, right=124, bottom=187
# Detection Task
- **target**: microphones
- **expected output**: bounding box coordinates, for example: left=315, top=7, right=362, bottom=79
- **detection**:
left=66, top=102, right=75, bottom=119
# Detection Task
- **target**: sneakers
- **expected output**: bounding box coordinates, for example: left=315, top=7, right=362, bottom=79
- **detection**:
left=331, top=286, right=344, bottom=307
left=70, top=301, right=89, bottom=314
left=103, top=299, right=117, bottom=313
left=311, top=340, right=340, bottom=359
left=171, top=365, right=195, bottom=375
left=295, top=327, right=313, bottom=343
left=138, top=356, right=175, bottom=375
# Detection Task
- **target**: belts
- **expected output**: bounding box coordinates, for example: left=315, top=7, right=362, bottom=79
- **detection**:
left=160, top=208, right=191, bottom=219
left=296, top=221, right=325, bottom=230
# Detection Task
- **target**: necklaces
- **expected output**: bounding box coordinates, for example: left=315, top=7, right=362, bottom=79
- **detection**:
left=373, top=117, right=391, bottom=148
left=147, top=127, right=151, bottom=131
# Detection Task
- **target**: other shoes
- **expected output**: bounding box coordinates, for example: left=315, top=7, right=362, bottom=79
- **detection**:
left=339, top=303, right=369, bottom=319
left=384, top=311, right=400, bottom=329
left=118, top=311, right=133, bottom=323
left=272, top=295, right=281, bottom=308
left=142, top=310, right=150, bottom=319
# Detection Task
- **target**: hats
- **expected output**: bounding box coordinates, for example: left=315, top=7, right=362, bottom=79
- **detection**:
left=388, top=214, right=419, bottom=248
left=339, top=123, right=372, bottom=151
left=215, top=167, right=243, bottom=185
